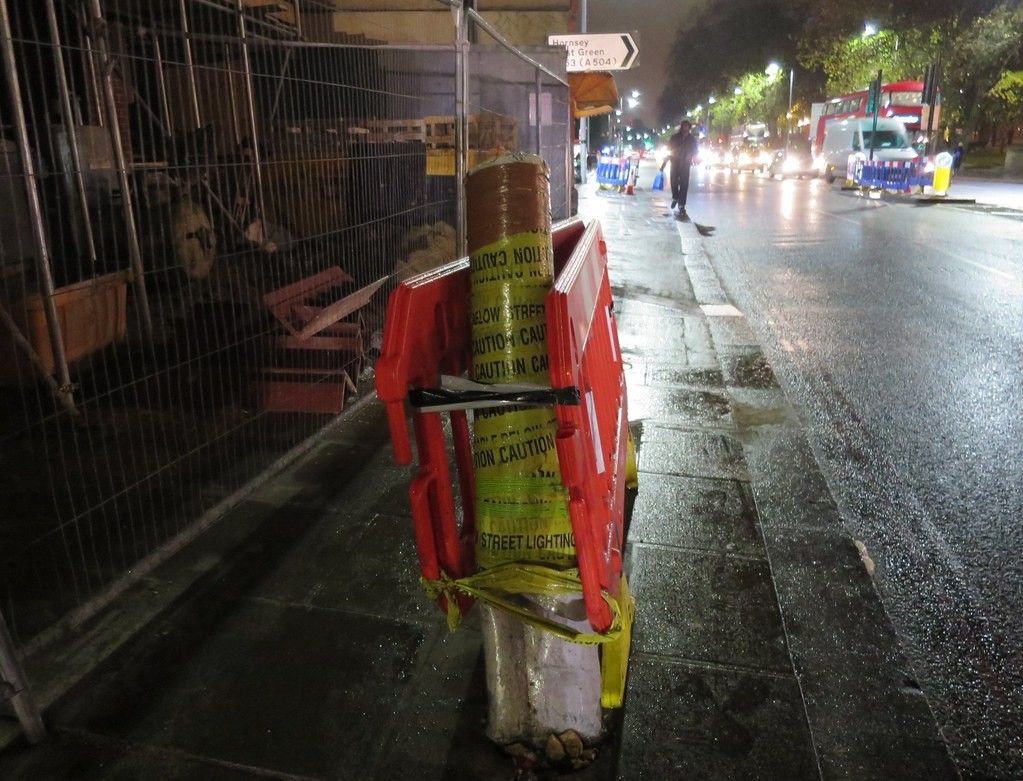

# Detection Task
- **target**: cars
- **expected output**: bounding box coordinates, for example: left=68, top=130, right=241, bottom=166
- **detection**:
left=724, top=145, right=812, bottom=181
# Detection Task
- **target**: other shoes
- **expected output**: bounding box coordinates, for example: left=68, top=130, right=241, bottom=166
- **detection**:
left=680, top=207, right=685, bottom=213
left=671, top=199, right=678, bottom=208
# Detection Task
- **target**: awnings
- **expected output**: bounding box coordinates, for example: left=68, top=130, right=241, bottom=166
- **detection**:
left=571, top=71, right=619, bottom=117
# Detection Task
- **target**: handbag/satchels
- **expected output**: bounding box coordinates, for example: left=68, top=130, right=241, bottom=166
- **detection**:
left=653, top=170, right=663, bottom=190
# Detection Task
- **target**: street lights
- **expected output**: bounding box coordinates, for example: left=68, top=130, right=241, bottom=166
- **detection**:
left=619, top=91, right=640, bottom=110
left=766, top=63, right=794, bottom=157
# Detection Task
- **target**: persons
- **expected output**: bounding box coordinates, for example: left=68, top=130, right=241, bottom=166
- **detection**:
left=667, top=120, right=697, bottom=212
left=954, top=142, right=963, bottom=173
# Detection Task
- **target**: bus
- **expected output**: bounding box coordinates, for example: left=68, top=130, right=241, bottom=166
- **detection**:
left=813, top=80, right=942, bottom=180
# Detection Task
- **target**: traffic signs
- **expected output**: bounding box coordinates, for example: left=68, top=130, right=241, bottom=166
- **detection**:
left=547, top=33, right=640, bottom=72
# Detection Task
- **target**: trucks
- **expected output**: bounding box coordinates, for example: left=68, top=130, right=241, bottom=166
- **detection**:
left=822, top=118, right=919, bottom=184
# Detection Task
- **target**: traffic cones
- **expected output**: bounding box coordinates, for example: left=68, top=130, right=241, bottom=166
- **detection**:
left=624, top=170, right=635, bottom=196
